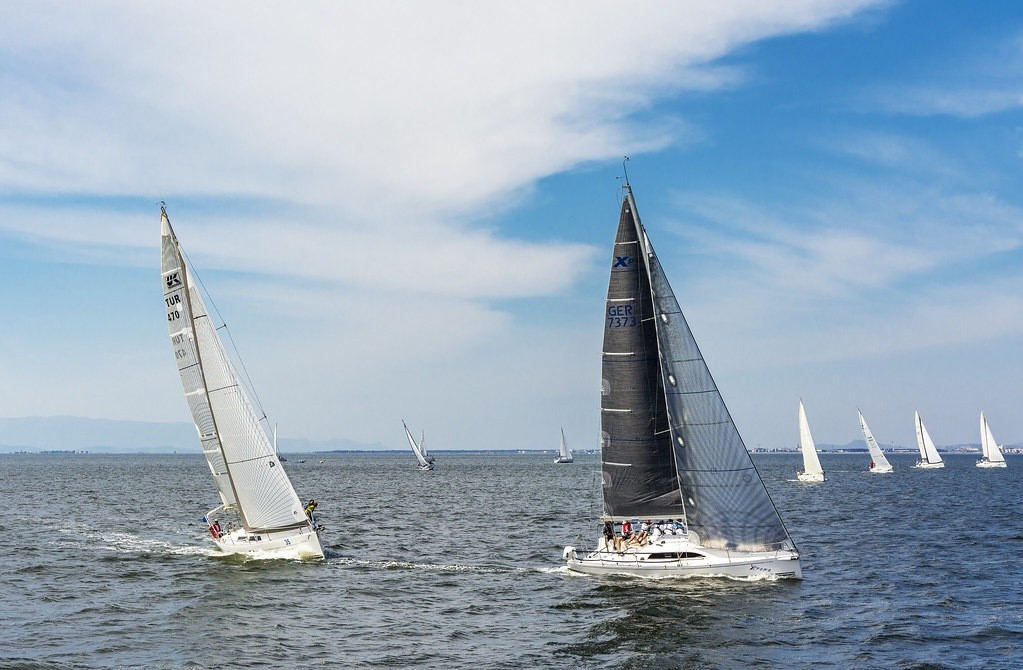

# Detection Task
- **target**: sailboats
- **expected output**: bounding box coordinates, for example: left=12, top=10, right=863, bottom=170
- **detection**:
left=796, top=397, right=826, bottom=482
left=401, top=420, right=435, bottom=471
left=157, top=202, right=327, bottom=563
left=857, top=408, right=895, bottom=475
left=975, top=409, right=1009, bottom=468
left=562, top=156, right=806, bottom=589
left=554, top=427, right=574, bottom=463
left=915, top=408, right=945, bottom=470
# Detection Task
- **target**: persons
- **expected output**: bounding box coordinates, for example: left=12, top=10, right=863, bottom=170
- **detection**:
left=303, top=500, right=318, bottom=520
left=208, top=519, right=223, bottom=537
left=603, top=520, right=685, bottom=551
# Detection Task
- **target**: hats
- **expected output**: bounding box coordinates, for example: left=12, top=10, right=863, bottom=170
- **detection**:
left=622, top=520, right=628, bottom=524
